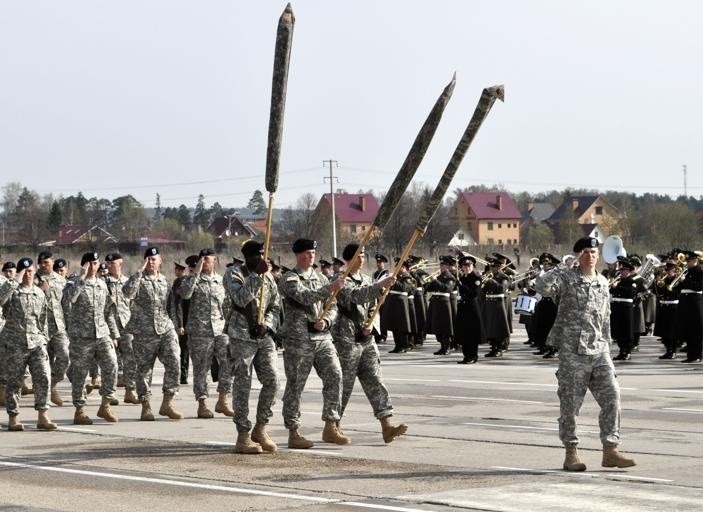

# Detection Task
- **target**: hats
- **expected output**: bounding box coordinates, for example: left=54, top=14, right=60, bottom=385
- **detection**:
left=98, top=264, right=108, bottom=272
left=143, top=247, right=159, bottom=259
left=199, top=248, right=215, bottom=259
left=291, top=240, right=317, bottom=254
left=319, top=260, right=331, bottom=268
left=81, top=252, right=99, bottom=266
left=617, top=248, right=700, bottom=270
left=227, top=240, right=280, bottom=270
left=342, top=243, right=359, bottom=260
left=574, top=237, right=600, bottom=253
left=373, top=252, right=560, bottom=267
left=1, top=251, right=65, bottom=271
left=333, top=257, right=345, bottom=267
left=174, top=263, right=185, bottom=270
left=185, top=255, right=199, bottom=266
left=104, top=253, right=122, bottom=261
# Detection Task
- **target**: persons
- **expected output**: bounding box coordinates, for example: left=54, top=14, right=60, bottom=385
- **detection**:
left=407, top=247, right=703, bottom=367
left=533, top=235, right=636, bottom=471
left=2, top=235, right=406, bottom=454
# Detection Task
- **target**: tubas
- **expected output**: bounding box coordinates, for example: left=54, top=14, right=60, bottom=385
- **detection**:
left=640, top=254, right=659, bottom=289
left=602, top=236, right=626, bottom=265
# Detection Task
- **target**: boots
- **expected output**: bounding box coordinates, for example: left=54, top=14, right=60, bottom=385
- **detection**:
left=563, top=449, right=586, bottom=471
left=235, top=416, right=407, bottom=453
left=602, top=446, right=635, bottom=467
left=1, top=376, right=234, bottom=431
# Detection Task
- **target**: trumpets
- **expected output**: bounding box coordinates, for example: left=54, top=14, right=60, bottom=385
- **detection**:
left=409, top=258, right=441, bottom=285
left=481, top=270, right=493, bottom=288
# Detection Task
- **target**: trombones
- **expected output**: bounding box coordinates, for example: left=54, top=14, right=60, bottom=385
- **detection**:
left=657, top=251, right=703, bottom=290
left=511, top=257, right=540, bottom=284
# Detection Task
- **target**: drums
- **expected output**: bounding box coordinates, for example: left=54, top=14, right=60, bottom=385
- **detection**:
left=514, top=294, right=537, bottom=316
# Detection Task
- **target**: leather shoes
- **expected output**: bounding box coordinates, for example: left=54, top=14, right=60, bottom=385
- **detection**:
left=659, top=352, right=702, bottom=362
left=524, top=340, right=558, bottom=359
left=612, top=345, right=638, bottom=360
left=376, top=335, right=509, bottom=364
